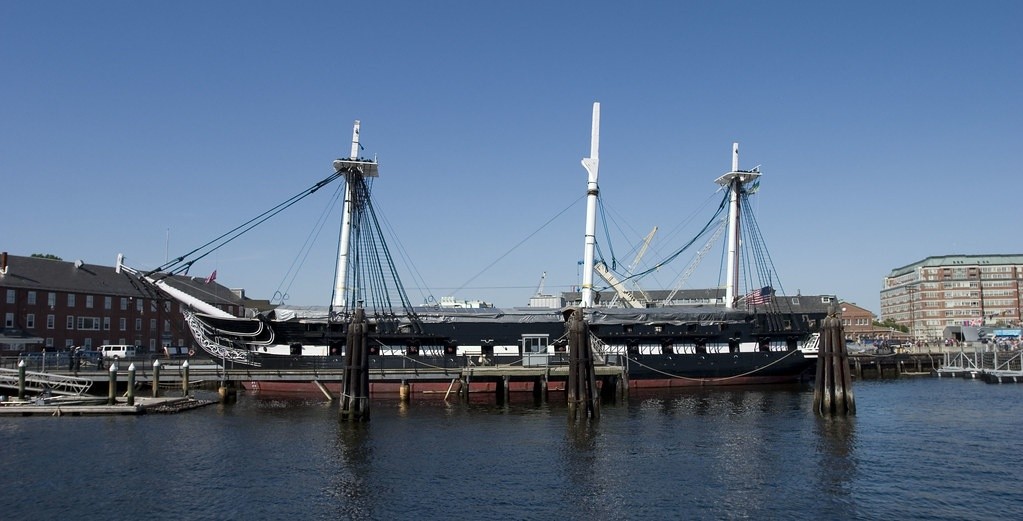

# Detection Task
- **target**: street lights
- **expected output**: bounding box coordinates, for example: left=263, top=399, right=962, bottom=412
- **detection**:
left=905, top=285, right=917, bottom=344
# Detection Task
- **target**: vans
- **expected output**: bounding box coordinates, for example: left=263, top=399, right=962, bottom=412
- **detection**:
left=98, top=345, right=136, bottom=359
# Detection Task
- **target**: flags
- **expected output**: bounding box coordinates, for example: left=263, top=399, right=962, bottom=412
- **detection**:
left=747, top=286, right=772, bottom=305
left=207, top=270, right=216, bottom=283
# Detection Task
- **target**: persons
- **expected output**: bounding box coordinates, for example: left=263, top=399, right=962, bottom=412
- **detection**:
left=68, top=346, right=81, bottom=372
left=906, top=339, right=929, bottom=347
left=98, top=348, right=105, bottom=369
left=945, top=338, right=960, bottom=347
left=478, top=354, right=491, bottom=365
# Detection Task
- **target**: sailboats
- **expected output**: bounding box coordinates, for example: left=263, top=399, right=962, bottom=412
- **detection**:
left=115, top=101, right=842, bottom=376
